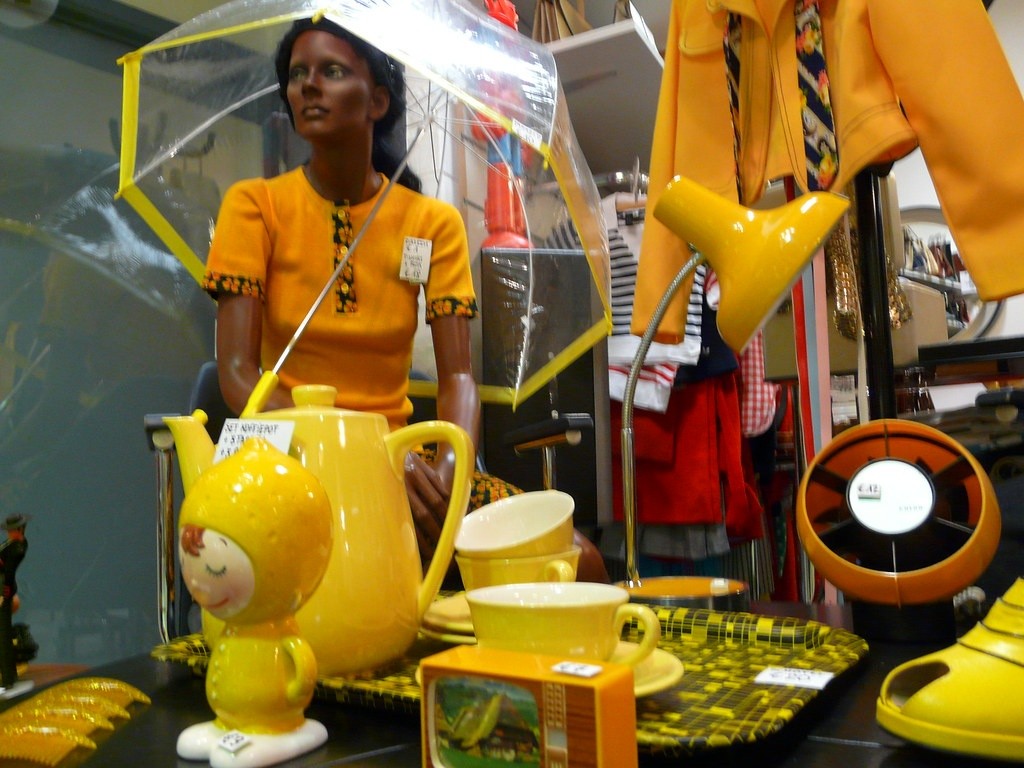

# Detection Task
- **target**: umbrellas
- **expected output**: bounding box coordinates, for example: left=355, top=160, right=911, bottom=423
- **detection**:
left=113, top=0, right=613, bottom=409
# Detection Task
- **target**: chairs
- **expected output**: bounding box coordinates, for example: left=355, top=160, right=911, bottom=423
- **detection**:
left=145, top=362, right=241, bottom=645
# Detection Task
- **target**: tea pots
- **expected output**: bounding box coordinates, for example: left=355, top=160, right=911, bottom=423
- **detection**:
left=160, top=382, right=472, bottom=677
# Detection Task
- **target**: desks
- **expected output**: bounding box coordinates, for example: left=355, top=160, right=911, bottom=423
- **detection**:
left=0, top=600, right=1024, bottom=768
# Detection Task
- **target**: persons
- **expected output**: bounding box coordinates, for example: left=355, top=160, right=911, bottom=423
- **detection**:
left=1, top=512, right=35, bottom=692
left=204, top=18, right=609, bottom=588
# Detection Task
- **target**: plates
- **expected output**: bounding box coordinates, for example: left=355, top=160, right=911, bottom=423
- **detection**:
left=420, top=590, right=481, bottom=646
left=415, top=641, right=682, bottom=702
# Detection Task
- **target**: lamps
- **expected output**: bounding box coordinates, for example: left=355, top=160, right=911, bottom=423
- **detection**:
left=608, top=174, right=850, bottom=603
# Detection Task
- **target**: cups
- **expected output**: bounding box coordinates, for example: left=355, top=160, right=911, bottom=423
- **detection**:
left=453, top=546, right=582, bottom=608
left=455, top=489, right=575, bottom=558
left=465, top=581, right=661, bottom=679
